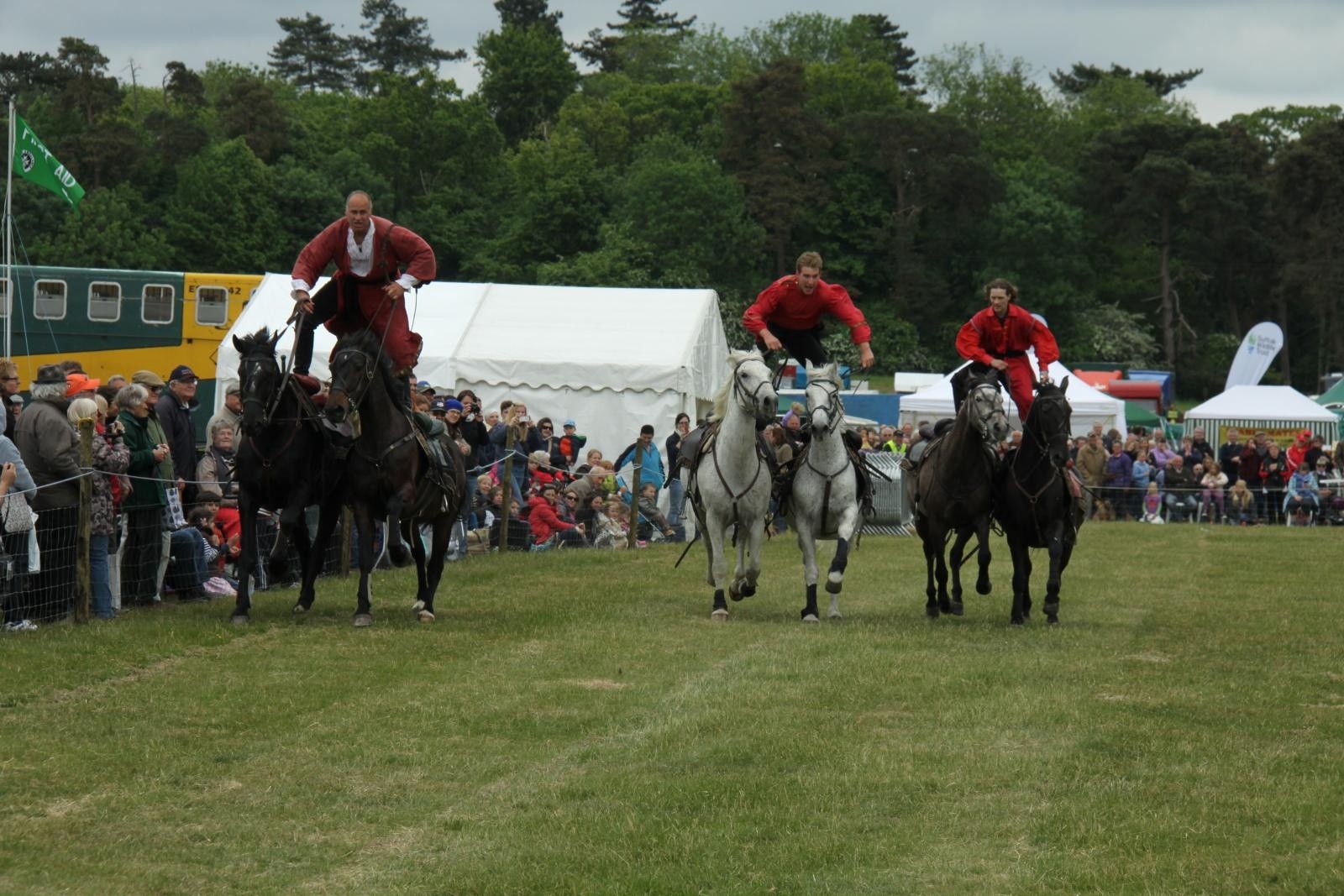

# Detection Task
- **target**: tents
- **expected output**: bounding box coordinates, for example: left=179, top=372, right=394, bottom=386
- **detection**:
left=213, top=270, right=732, bottom=540
left=899, top=313, right=1344, bottom=449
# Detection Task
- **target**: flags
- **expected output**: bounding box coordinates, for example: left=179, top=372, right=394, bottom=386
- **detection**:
left=10, top=109, right=85, bottom=220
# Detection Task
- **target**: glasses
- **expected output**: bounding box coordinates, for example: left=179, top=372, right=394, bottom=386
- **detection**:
left=1317, top=464, right=1326, bottom=468
left=566, top=495, right=579, bottom=502
left=540, top=425, right=554, bottom=430
left=2, top=377, right=19, bottom=383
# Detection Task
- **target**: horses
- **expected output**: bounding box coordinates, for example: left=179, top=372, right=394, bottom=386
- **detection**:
left=685, top=345, right=780, bottom=623
left=293, top=319, right=469, bottom=629
left=992, top=375, right=1088, bottom=627
left=901, top=368, right=1011, bottom=623
left=791, top=355, right=872, bottom=624
left=231, top=327, right=361, bottom=629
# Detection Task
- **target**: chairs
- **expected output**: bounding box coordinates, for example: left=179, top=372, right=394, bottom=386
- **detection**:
left=1164, top=500, right=1316, bottom=528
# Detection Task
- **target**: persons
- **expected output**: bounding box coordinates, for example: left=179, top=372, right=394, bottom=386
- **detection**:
left=399, top=374, right=710, bottom=549
left=950, top=279, right=1059, bottom=427
left=291, top=190, right=436, bottom=388
left=743, top=251, right=874, bottom=368
left=1007, top=422, right=1344, bottom=527
left=763, top=401, right=933, bottom=534
left=0, top=362, right=360, bottom=624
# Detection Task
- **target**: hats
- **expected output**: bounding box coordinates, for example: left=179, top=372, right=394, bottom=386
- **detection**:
left=564, top=421, right=575, bottom=426
left=10, top=394, right=24, bottom=403
left=226, top=383, right=241, bottom=394
left=32, top=364, right=65, bottom=384
left=415, top=381, right=463, bottom=412
left=1297, top=429, right=1313, bottom=442
left=169, top=365, right=199, bottom=381
left=132, top=370, right=166, bottom=387
left=65, top=374, right=101, bottom=396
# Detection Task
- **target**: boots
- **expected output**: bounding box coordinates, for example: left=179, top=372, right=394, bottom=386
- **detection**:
left=446, top=519, right=468, bottom=561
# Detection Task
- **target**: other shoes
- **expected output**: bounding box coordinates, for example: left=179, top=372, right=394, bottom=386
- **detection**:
left=397, top=374, right=412, bottom=408
left=1080, top=515, right=1343, bottom=525
left=5, top=621, right=38, bottom=633
left=662, top=529, right=677, bottom=537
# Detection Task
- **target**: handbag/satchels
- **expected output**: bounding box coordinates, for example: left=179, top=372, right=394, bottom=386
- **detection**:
left=0, top=493, right=33, bottom=532
left=156, top=487, right=187, bottom=532
